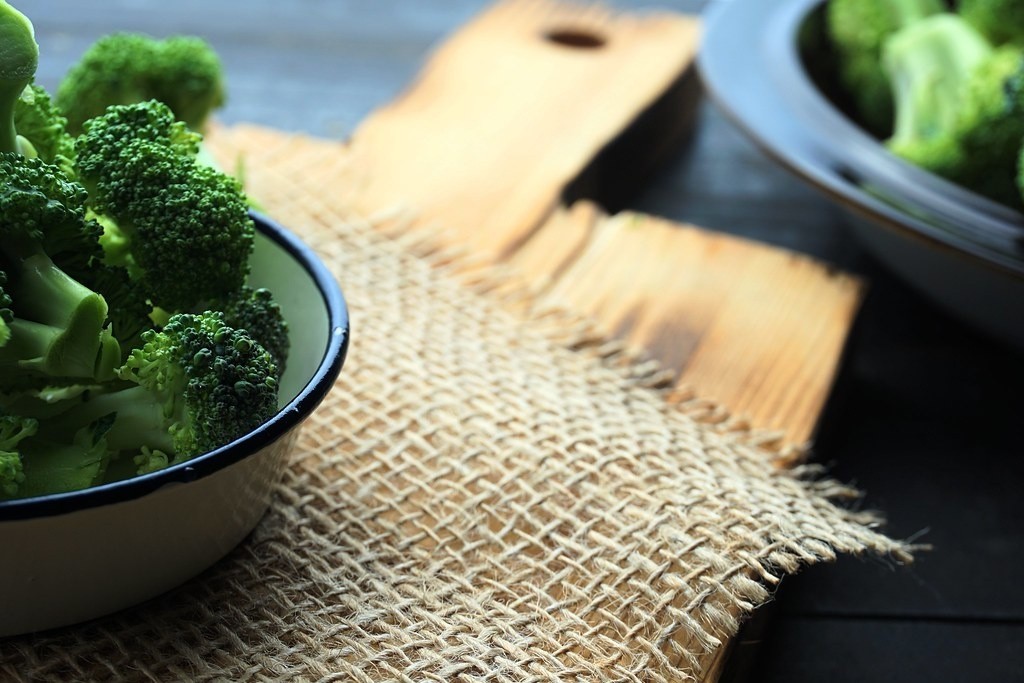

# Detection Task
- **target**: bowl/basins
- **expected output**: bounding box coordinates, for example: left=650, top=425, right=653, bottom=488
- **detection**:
left=691, top=0, right=1023, bottom=366
left=0, top=200, right=350, bottom=646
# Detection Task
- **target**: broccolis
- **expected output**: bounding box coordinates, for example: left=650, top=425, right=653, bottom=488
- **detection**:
left=807, top=0, right=1024, bottom=218
left=0, top=1, right=290, bottom=503
